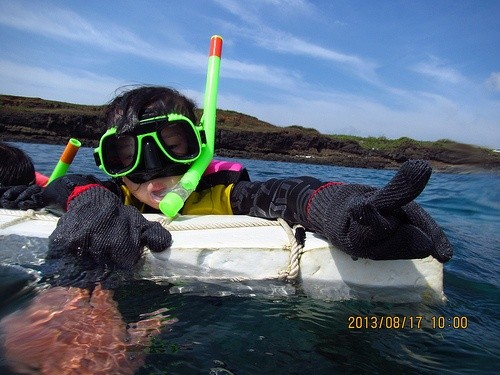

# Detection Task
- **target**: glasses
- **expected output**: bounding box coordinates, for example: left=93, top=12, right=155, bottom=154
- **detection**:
left=95, top=114, right=205, bottom=184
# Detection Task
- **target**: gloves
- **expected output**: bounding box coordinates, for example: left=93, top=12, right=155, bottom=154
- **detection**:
left=50, top=187, right=172, bottom=270
left=1, top=185, right=46, bottom=208
left=307, top=159, right=452, bottom=262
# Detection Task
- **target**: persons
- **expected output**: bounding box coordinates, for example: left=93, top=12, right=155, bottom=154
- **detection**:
left=40, top=81, right=453, bottom=304
left=0, top=143, right=36, bottom=191
